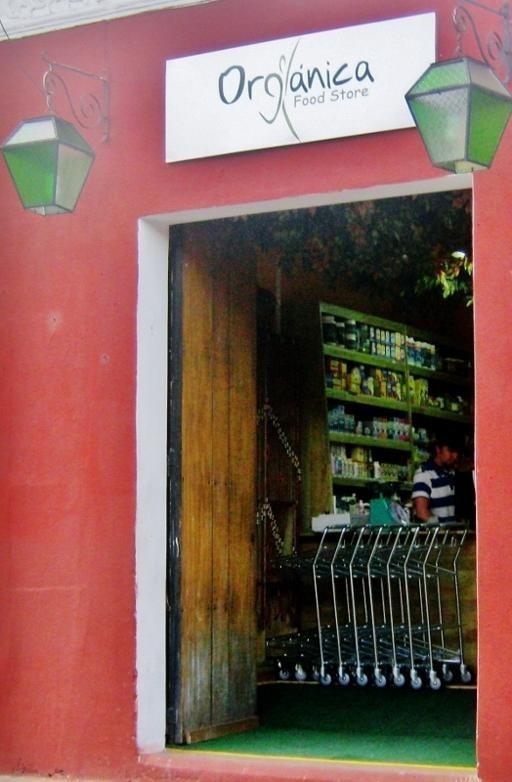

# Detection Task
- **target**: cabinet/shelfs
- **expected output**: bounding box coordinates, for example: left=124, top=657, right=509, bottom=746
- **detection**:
left=294, top=296, right=475, bottom=534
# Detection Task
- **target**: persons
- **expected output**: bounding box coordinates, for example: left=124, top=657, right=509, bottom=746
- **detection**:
left=411, top=429, right=460, bottom=546
left=452, top=445, right=475, bottom=482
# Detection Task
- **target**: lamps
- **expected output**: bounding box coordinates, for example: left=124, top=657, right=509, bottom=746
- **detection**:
left=2, top=58, right=110, bottom=217
left=403, top=7, right=512, bottom=173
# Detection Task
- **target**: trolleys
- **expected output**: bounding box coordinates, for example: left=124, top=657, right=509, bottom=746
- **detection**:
left=257, top=523, right=476, bottom=698
